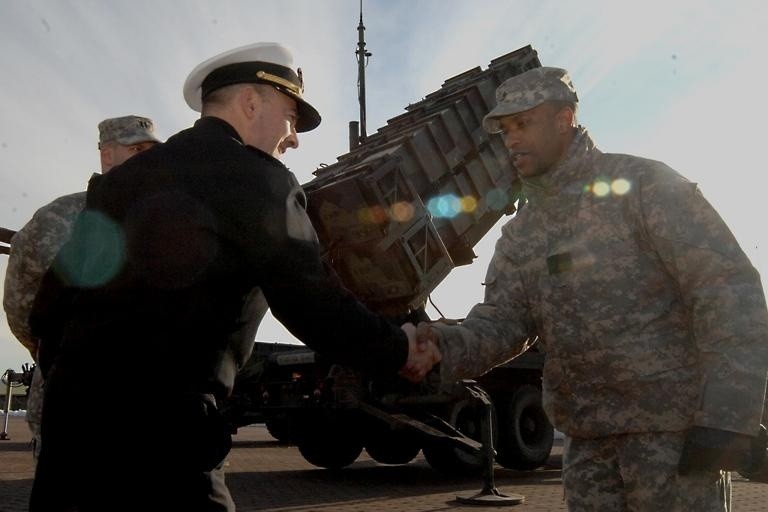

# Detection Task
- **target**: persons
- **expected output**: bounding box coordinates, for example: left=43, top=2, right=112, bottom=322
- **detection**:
left=2, top=115, right=161, bottom=464
left=405, top=68, right=768, bottom=512
left=23, top=42, right=443, bottom=512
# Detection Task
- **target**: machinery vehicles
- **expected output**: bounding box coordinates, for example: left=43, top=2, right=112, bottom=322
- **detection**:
left=263, top=35, right=767, bottom=506
left=1, top=339, right=301, bottom=447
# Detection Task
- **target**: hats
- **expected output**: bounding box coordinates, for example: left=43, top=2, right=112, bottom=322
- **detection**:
left=181, top=38, right=323, bottom=134
left=96, top=114, right=164, bottom=151
left=480, top=66, right=581, bottom=136
